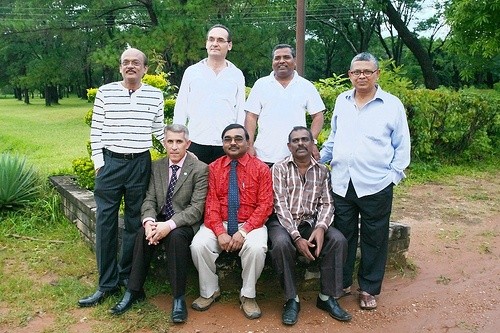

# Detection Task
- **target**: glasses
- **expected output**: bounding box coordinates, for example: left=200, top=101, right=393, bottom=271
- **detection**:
left=207, top=38, right=228, bottom=44
left=350, top=70, right=376, bottom=76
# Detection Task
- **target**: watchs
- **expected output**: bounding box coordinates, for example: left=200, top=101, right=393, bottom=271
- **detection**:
left=238, top=229, right=246, bottom=238
left=313, top=138, right=318, bottom=144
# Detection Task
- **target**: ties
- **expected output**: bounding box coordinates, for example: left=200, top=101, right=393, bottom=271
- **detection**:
left=227, top=161, right=238, bottom=235
left=165, top=165, right=179, bottom=220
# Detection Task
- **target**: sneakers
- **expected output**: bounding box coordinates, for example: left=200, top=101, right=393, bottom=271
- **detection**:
left=239, top=293, right=261, bottom=319
left=192, top=290, right=220, bottom=311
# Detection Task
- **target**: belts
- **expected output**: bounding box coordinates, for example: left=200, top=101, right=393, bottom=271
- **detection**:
left=104, top=147, right=147, bottom=159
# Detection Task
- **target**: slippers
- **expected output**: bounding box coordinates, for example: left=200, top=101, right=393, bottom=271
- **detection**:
left=333, top=288, right=351, bottom=299
left=359, top=293, right=377, bottom=310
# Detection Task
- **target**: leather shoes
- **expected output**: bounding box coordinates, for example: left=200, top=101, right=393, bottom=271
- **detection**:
left=171, top=297, right=186, bottom=322
left=282, top=299, right=301, bottom=324
left=77, top=290, right=113, bottom=306
left=110, top=288, right=145, bottom=313
left=316, top=293, right=352, bottom=320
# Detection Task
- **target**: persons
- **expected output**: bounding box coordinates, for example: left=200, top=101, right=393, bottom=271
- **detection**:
left=110, top=124, right=209, bottom=323
left=190, top=124, right=274, bottom=319
left=173, top=24, right=246, bottom=165
left=270, top=127, right=352, bottom=325
left=318, top=52, right=411, bottom=309
left=243, top=44, right=327, bottom=167
left=78, top=48, right=198, bottom=315
